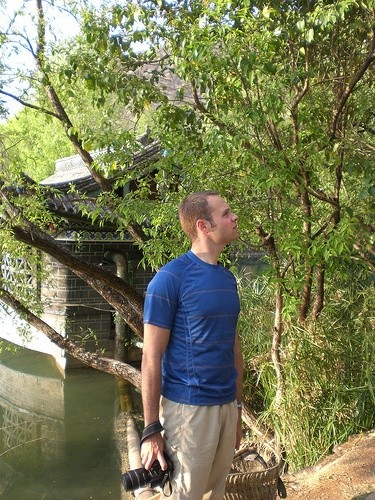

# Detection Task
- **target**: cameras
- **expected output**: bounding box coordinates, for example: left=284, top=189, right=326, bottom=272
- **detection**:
left=120, top=449, right=174, bottom=492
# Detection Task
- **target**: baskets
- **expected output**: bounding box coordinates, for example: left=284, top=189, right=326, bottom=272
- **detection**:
left=222, top=454, right=281, bottom=500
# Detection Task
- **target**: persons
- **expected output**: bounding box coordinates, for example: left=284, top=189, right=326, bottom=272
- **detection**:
left=139, top=192, right=244, bottom=500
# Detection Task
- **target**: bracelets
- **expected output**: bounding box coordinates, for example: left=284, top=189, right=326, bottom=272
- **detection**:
left=238, top=401, right=244, bottom=409
